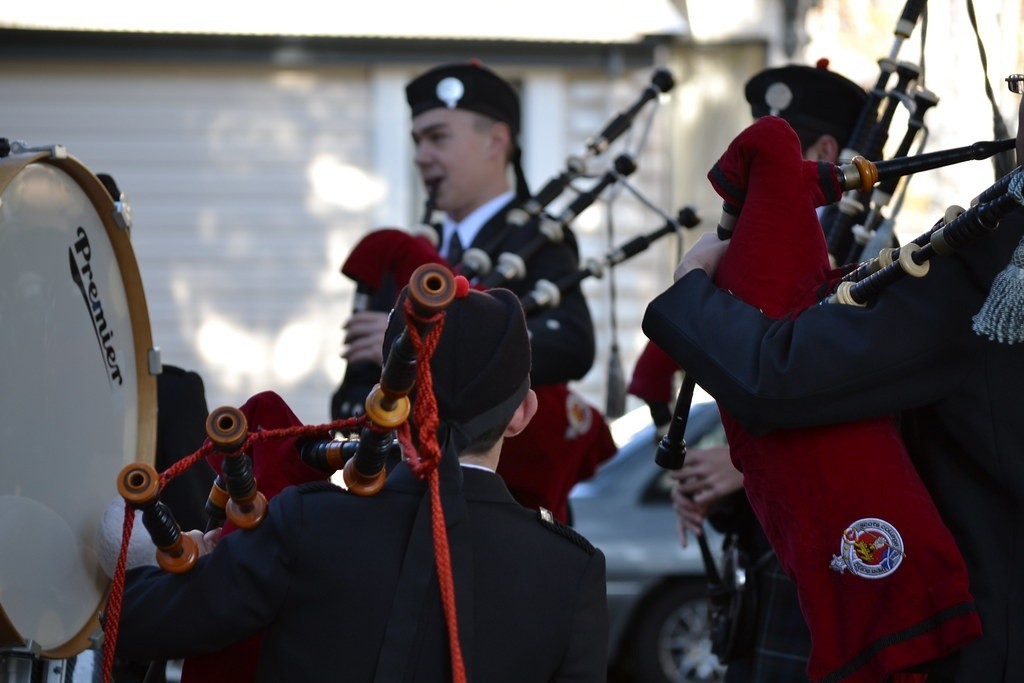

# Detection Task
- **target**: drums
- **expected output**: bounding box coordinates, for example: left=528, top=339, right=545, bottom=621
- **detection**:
left=1, top=138, right=165, bottom=662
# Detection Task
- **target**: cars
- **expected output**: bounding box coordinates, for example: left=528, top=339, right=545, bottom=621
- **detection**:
left=543, top=381, right=750, bottom=683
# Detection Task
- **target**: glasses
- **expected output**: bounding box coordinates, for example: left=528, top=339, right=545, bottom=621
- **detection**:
left=1005, top=74, right=1024, bottom=94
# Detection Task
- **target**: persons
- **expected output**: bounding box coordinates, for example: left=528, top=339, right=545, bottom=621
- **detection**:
left=674, top=61, right=899, bottom=683
left=328, top=61, right=596, bottom=437
left=641, top=138, right=1023, bottom=683
left=101, top=278, right=612, bottom=683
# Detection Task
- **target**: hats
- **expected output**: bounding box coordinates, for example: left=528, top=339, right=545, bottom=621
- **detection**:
left=406, top=62, right=520, bottom=132
left=382, top=283, right=531, bottom=446
left=745, top=59, right=866, bottom=128
